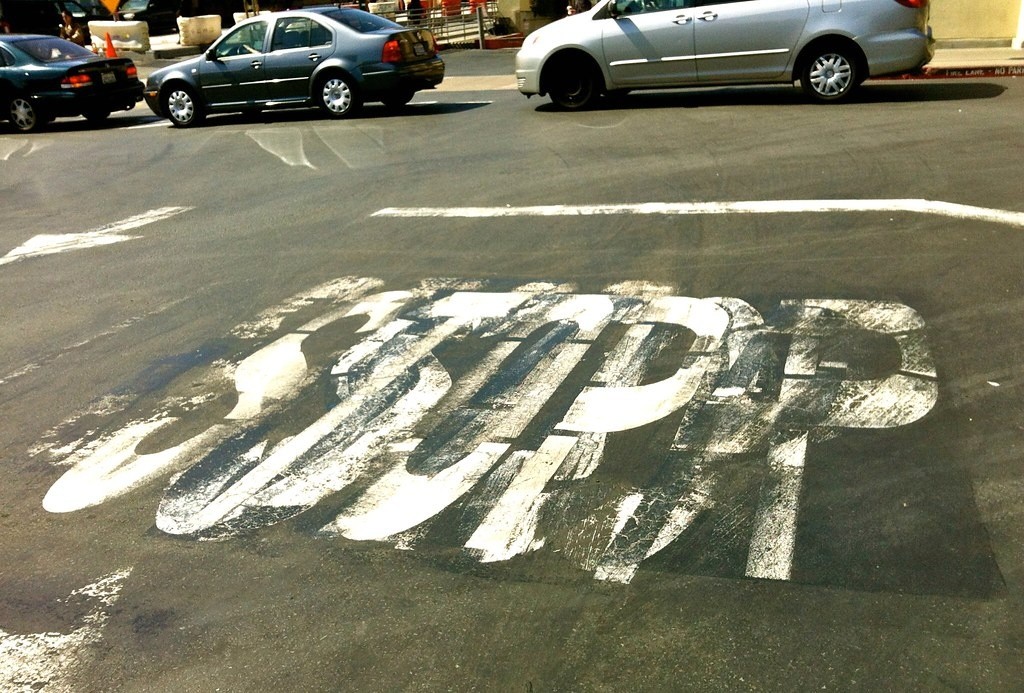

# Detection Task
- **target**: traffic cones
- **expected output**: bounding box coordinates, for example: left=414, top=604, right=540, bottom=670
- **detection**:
left=105, top=32, right=117, bottom=58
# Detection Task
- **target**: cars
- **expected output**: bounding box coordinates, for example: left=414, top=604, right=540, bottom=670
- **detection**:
left=0, top=35, right=146, bottom=133
left=143, top=6, right=445, bottom=128
left=113, top=0, right=186, bottom=33
left=515, top=0, right=937, bottom=111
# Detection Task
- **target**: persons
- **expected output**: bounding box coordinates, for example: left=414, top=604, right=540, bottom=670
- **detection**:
left=60, top=10, right=84, bottom=48
left=567, top=0, right=592, bottom=15
